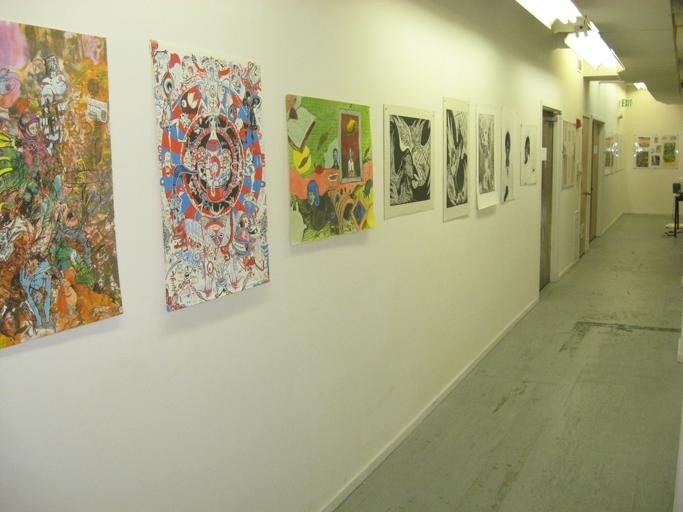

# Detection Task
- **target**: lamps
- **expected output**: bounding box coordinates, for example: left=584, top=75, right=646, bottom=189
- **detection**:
left=513, top=0, right=621, bottom=69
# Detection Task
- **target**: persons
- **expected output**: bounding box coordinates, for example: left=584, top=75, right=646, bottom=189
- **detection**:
left=501, top=130, right=515, bottom=201
left=520, top=135, right=535, bottom=185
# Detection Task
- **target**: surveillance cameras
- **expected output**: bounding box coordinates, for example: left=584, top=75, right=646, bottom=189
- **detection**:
left=576, top=16, right=586, bottom=32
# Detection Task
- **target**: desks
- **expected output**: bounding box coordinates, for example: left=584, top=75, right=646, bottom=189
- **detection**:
left=674, top=194, right=683, bottom=236
